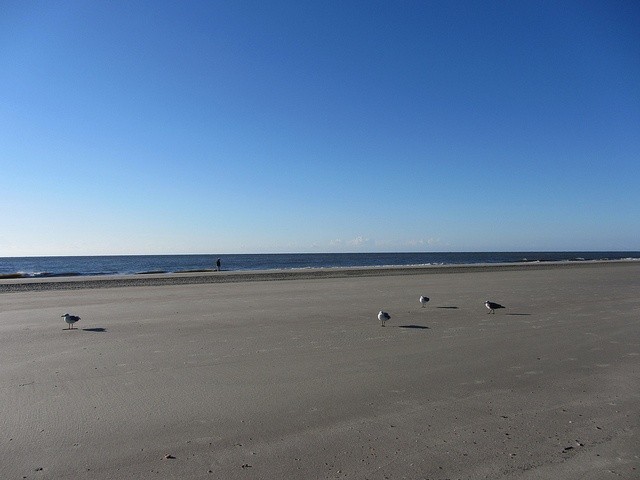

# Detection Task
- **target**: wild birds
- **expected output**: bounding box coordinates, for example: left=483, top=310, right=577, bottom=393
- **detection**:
left=60, top=312, right=81, bottom=330
left=377, top=310, right=392, bottom=328
left=483, top=300, right=506, bottom=314
left=419, top=295, right=431, bottom=308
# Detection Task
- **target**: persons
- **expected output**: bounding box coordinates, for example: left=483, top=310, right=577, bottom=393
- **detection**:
left=216, top=258, right=221, bottom=272
